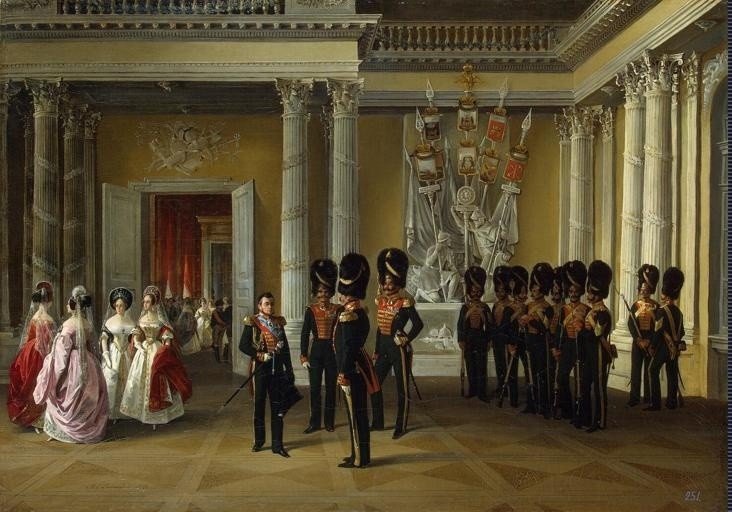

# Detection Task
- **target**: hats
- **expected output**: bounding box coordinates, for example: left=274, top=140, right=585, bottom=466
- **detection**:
left=69, top=284, right=92, bottom=309
left=280, top=371, right=305, bottom=409
left=108, top=285, right=133, bottom=312
left=461, top=258, right=683, bottom=301
left=140, top=284, right=162, bottom=305
left=338, top=252, right=367, bottom=300
left=32, top=281, right=56, bottom=304
left=311, top=257, right=338, bottom=299
left=376, top=247, right=407, bottom=288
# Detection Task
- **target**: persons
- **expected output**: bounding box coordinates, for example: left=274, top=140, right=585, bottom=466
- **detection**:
left=644, top=293, right=684, bottom=411
left=457, top=284, right=613, bottom=434
left=368, top=274, right=424, bottom=438
left=331, top=293, right=381, bottom=468
left=240, top=293, right=294, bottom=458
left=415, top=233, right=458, bottom=304
left=7, top=281, right=232, bottom=444
left=451, top=205, right=509, bottom=302
left=300, top=286, right=345, bottom=434
left=628, top=287, right=660, bottom=407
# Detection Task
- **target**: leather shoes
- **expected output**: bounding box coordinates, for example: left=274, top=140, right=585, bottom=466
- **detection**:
left=250, top=421, right=409, bottom=471
left=464, top=390, right=680, bottom=434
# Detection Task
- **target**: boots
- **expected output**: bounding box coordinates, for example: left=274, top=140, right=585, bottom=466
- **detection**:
left=213, top=341, right=228, bottom=361
